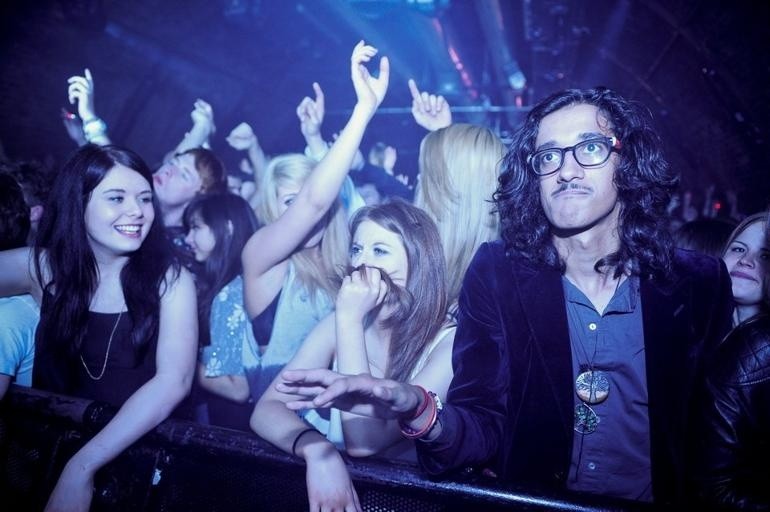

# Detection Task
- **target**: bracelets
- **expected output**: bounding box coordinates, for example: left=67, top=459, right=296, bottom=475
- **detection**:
left=393, top=384, right=427, bottom=421
left=397, top=391, right=443, bottom=439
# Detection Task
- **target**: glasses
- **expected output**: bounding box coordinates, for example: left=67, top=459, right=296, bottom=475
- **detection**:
left=528, top=138, right=619, bottom=177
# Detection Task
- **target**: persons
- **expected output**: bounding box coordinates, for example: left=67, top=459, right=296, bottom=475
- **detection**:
left=275, top=89, right=737, bottom=512
left=655, top=149, right=769, bottom=512
left=0, top=39, right=510, bottom=512
